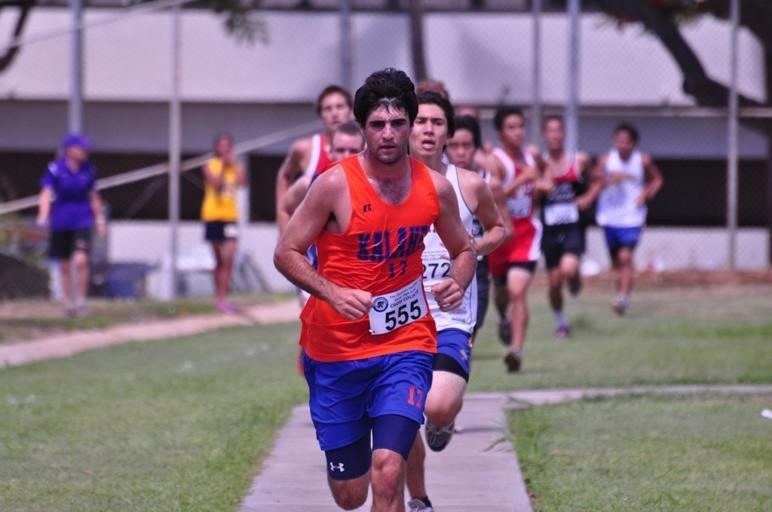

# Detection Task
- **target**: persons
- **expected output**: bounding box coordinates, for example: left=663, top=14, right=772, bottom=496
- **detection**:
left=414, top=79, right=553, bottom=372
left=32, top=132, right=109, bottom=319
left=403, top=88, right=510, bottom=512
left=537, top=115, right=663, bottom=336
left=273, top=64, right=480, bottom=512
left=198, top=129, right=247, bottom=319
left=276, top=84, right=367, bottom=311
